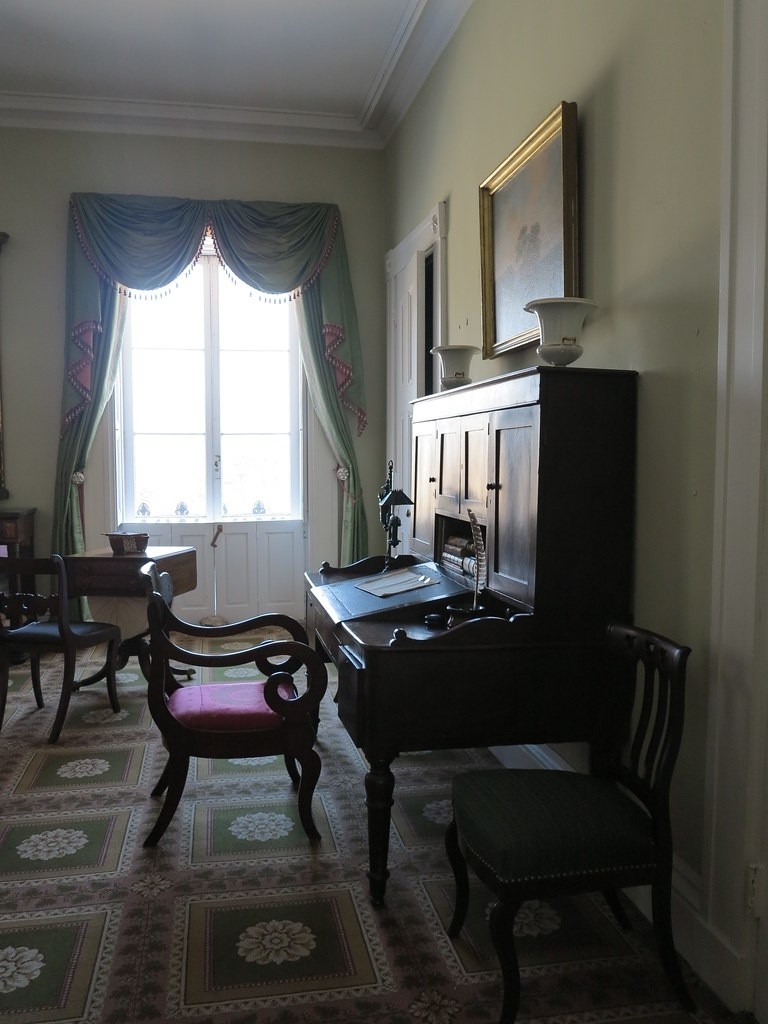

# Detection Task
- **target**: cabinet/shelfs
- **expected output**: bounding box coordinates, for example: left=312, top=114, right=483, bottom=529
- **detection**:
left=303, top=365, right=637, bottom=913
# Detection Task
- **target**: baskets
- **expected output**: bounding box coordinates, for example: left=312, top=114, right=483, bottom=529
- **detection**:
left=105, top=531, right=149, bottom=554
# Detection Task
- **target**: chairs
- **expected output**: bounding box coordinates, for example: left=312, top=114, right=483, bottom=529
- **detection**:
left=135, top=561, right=329, bottom=852
left=0, top=554, right=121, bottom=744
left=443, top=624, right=692, bottom=1024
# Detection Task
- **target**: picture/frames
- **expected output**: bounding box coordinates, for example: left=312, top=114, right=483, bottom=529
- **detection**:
left=478, top=100, right=581, bottom=361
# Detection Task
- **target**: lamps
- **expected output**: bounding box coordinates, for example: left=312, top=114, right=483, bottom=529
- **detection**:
left=377, top=459, right=415, bottom=575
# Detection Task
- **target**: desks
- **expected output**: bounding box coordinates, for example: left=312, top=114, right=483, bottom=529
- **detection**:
left=0, top=507, right=37, bottom=666
left=63, top=544, right=197, bottom=705
